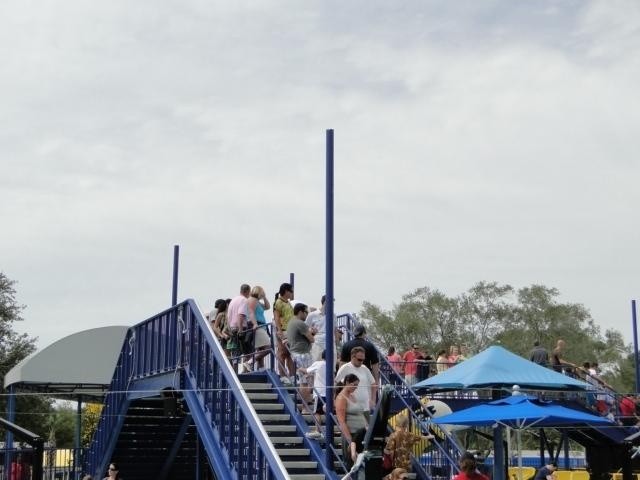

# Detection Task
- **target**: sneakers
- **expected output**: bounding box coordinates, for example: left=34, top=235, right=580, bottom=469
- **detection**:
left=242, top=362, right=252, bottom=374
left=305, top=431, right=325, bottom=441
left=279, top=375, right=300, bottom=385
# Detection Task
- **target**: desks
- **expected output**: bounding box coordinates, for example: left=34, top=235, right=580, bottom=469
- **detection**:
left=553, top=470, right=571, bottom=480
left=508, top=467, right=536, bottom=480
left=571, top=471, right=590, bottom=480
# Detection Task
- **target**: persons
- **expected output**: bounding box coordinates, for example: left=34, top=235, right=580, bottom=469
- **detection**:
left=101, top=461, right=122, bottom=480
left=529, top=337, right=639, bottom=426
left=206, top=282, right=437, bottom=479
left=437, top=344, right=490, bottom=399
left=10, top=451, right=31, bottom=480
left=453, top=452, right=485, bottom=480
left=533, top=462, right=558, bottom=479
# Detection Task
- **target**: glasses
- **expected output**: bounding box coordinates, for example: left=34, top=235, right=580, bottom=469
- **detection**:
left=354, top=356, right=366, bottom=362
